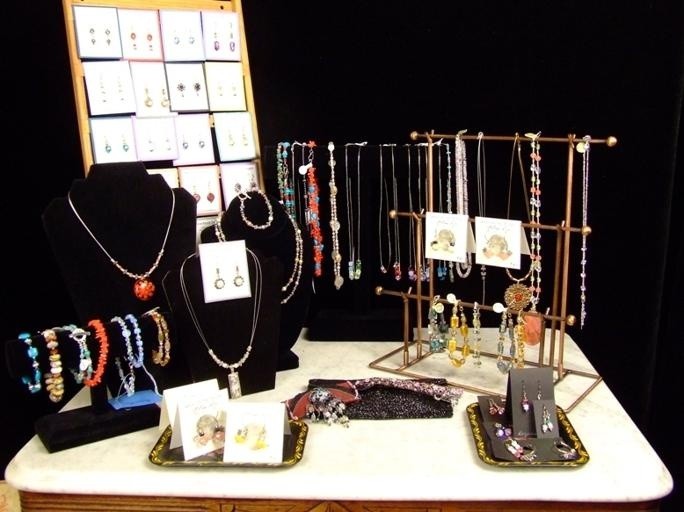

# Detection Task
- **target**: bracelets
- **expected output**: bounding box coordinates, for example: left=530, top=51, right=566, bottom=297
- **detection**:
left=428, top=293, right=529, bottom=375
left=18, top=307, right=172, bottom=403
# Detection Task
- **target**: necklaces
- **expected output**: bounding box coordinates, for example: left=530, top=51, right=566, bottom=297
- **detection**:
left=211, top=210, right=303, bottom=305
left=66, top=183, right=176, bottom=302
left=235, top=185, right=274, bottom=229
left=178, top=247, right=263, bottom=399
left=276, top=129, right=592, bottom=330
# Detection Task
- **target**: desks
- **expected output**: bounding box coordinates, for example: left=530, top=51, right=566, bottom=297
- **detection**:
left=4, top=326, right=675, bottom=512
